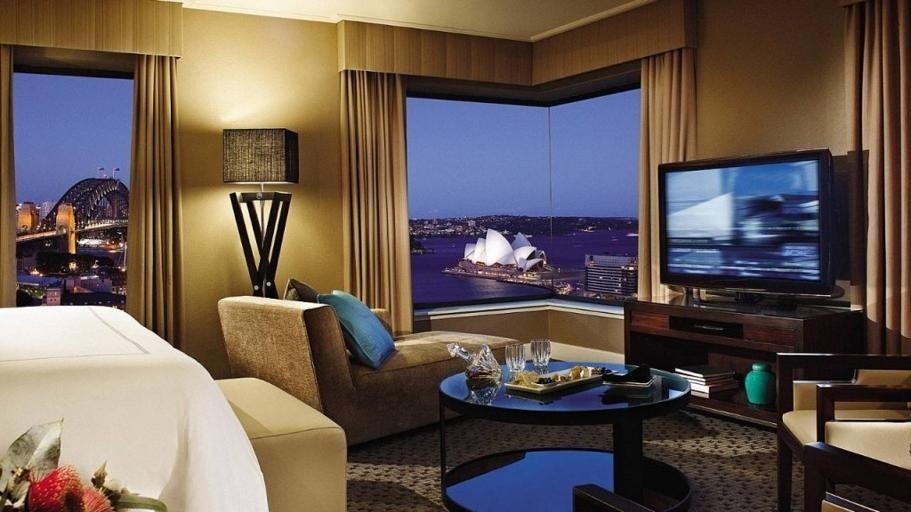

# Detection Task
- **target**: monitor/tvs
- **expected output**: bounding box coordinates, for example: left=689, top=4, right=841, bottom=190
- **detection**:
left=658, top=146, right=834, bottom=307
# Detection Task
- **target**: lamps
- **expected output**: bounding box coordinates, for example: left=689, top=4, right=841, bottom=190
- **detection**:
left=219, top=127, right=302, bottom=299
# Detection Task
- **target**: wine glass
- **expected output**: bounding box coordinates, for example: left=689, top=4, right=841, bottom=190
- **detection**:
left=532, top=339, right=551, bottom=372
left=501, top=339, right=527, bottom=372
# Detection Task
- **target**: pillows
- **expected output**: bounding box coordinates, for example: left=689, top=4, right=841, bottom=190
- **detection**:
left=313, top=286, right=397, bottom=371
left=283, top=277, right=394, bottom=349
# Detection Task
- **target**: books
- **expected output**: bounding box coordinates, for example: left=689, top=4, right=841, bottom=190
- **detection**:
left=673, top=364, right=739, bottom=399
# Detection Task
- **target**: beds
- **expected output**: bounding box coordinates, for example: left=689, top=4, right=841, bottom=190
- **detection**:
left=0, top=307, right=271, bottom=510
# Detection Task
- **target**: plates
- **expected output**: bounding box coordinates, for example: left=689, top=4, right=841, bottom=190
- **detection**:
left=505, top=364, right=613, bottom=393
left=504, top=381, right=602, bottom=405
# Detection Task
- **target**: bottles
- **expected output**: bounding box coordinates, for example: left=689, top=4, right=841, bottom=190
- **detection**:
left=746, top=363, right=777, bottom=405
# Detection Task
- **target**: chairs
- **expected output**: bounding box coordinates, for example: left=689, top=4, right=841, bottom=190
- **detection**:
left=215, top=293, right=523, bottom=447
left=805, top=418, right=911, bottom=511
left=776, top=348, right=911, bottom=510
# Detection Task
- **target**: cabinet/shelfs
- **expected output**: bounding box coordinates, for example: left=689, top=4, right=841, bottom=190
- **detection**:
left=621, top=297, right=870, bottom=433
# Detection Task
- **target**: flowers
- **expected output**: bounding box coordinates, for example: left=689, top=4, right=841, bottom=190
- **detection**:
left=0, top=414, right=171, bottom=511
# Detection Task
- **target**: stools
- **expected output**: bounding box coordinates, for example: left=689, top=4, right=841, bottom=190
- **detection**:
left=205, top=374, right=352, bottom=511
